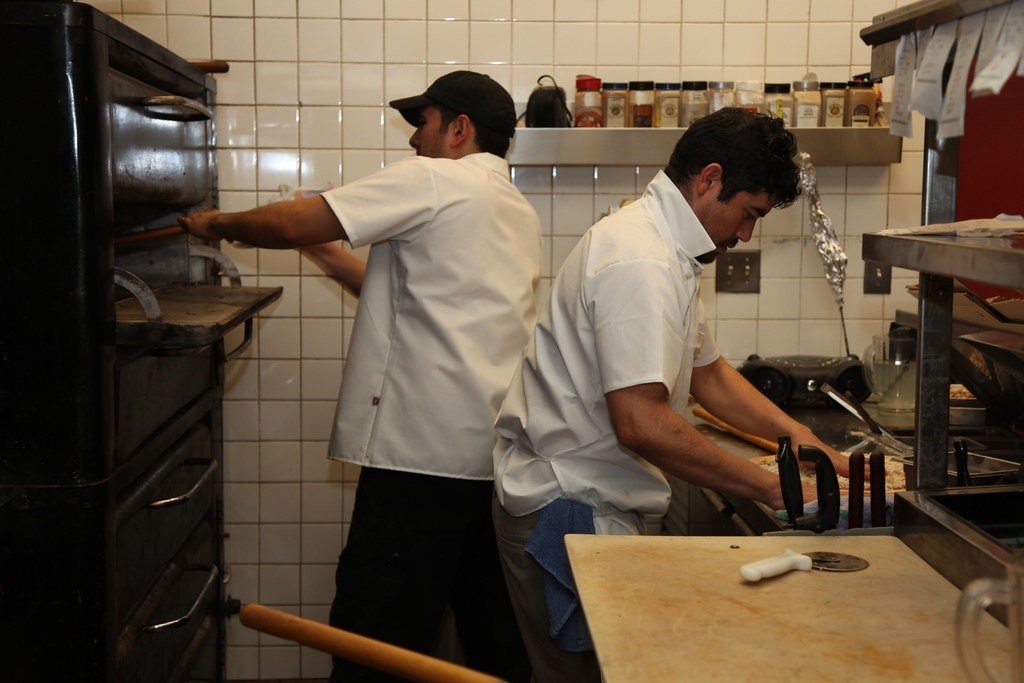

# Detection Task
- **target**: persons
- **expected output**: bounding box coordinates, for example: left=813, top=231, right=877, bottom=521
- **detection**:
left=492, top=107, right=885, bottom=683
left=178, top=71, right=543, bottom=683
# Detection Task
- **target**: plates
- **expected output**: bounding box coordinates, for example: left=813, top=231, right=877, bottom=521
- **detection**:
left=947, top=406, right=985, bottom=426
left=949, top=384, right=978, bottom=407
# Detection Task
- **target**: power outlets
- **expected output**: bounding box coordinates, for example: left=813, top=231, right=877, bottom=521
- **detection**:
left=714, top=248, right=762, bottom=295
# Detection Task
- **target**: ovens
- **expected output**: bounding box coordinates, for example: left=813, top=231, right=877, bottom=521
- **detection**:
left=0, top=1, right=227, bottom=683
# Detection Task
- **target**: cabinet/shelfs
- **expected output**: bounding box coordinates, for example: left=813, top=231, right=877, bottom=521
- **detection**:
left=860, top=0, right=1024, bottom=628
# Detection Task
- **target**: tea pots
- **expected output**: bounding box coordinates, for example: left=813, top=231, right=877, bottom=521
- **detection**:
left=861, top=320, right=919, bottom=432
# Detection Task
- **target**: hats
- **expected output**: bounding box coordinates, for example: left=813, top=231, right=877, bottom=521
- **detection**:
left=388, top=69, right=516, bottom=140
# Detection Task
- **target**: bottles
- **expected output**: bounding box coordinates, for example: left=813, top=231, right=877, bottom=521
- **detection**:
left=601, top=82, right=628, bottom=128
left=655, top=80, right=877, bottom=128
left=572, top=74, right=603, bottom=128
left=628, top=81, right=654, bottom=127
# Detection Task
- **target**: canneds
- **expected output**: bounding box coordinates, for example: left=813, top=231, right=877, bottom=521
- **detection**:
left=574, top=77, right=877, bottom=130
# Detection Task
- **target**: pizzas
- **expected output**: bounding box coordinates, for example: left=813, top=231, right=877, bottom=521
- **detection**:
left=741, top=454, right=910, bottom=494
